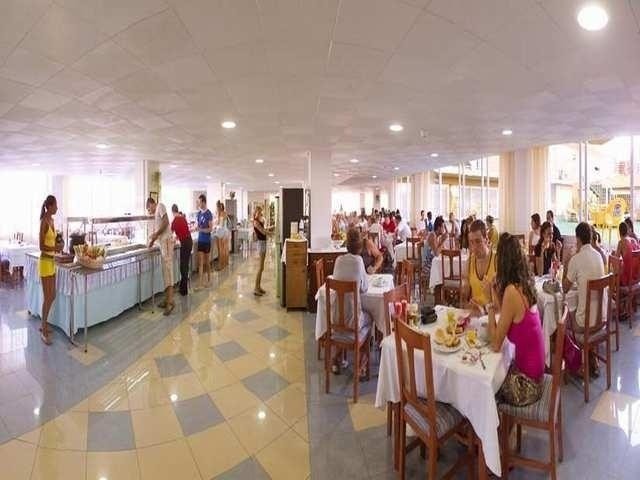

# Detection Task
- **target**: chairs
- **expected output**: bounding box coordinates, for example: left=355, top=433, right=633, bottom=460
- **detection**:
left=565, top=273, right=616, bottom=402
left=497, top=301, right=571, bottom=479
left=309, top=233, right=543, bottom=401
left=530, top=272, right=566, bottom=370
left=608, top=256, right=623, bottom=352
left=618, top=249, right=640, bottom=330
left=393, top=315, right=475, bottom=480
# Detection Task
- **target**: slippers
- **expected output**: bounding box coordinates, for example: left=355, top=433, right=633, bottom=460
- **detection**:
left=163, top=301, right=175, bottom=315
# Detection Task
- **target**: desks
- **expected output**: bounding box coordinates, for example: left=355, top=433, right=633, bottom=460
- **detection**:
left=23, top=232, right=228, bottom=344
left=0, top=236, right=31, bottom=278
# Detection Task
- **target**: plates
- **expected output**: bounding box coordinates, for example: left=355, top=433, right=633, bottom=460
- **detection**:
left=432, top=339, right=464, bottom=353
left=373, top=283, right=386, bottom=287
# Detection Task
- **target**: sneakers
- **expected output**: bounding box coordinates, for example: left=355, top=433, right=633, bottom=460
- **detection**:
left=332, top=360, right=349, bottom=373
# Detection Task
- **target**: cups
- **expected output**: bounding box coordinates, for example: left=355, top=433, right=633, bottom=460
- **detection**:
left=480, top=323, right=488, bottom=338
left=373, top=276, right=385, bottom=283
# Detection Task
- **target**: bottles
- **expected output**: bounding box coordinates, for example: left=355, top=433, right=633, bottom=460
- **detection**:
left=393, top=296, right=421, bottom=330
left=299, top=219, right=304, bottom=234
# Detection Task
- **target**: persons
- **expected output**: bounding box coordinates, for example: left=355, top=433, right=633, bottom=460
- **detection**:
left=146, top=198, right=177, bottom=315
left=487, top=233, right=545, bottom=407
left=38, top=195, right=64, bottom=345
left=215, top=199, right=231, bottom=271
left=170, top=204, right=193, bottom=296
left=194, top=194, right=213, bottom=289
left=331, top=227, right=367, bottom=382
left=562, top=222, right=609, bottom=380
left=252, top=206, right=275, bottom=296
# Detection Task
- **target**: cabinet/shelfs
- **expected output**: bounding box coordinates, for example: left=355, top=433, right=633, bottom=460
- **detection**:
left=65, top=215, right=156, bottom=353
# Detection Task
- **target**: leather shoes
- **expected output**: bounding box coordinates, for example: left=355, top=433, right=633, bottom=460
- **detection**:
left=41, top=333, right=52, bottom=345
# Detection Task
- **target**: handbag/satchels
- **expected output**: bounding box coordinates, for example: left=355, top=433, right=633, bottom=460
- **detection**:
left=563, top=302, right=582, bottom=372
